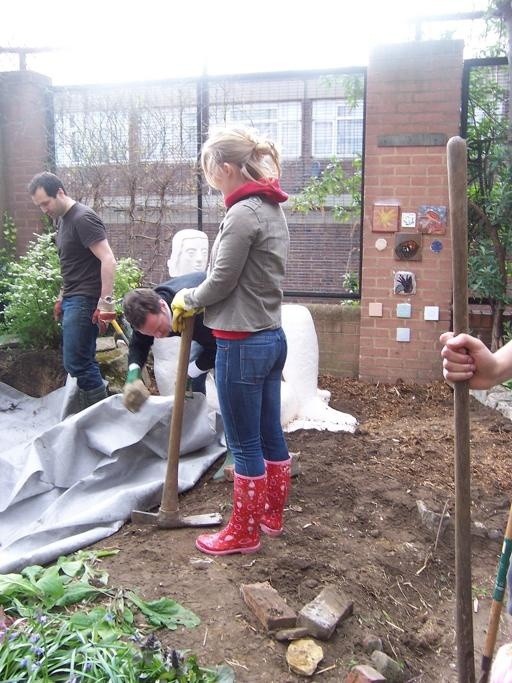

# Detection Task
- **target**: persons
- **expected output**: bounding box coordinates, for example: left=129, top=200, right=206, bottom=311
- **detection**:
left=27, top=170, right=117, bottom=408
left=172, top=121, right=292, bottom=556
left=439, top=332, right=512, bottom=683
left=123, top=270, right=235, bottom=481
left=166, top=227, right=210, bottom=278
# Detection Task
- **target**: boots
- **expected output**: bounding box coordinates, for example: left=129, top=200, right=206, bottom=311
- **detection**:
left=195, top=459, right=267, bottom=556
left=79, top=382, right=107, bottom=412
left=259, top=452, right=293, bottom=537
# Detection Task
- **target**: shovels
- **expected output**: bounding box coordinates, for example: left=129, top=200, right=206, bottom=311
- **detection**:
left=111, top=319, right=151, bottom=389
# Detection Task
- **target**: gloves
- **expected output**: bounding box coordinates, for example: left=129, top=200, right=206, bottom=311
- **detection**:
left=122, top=367, right=151, bottom=414
left=92, top=298, right=117, bottom=336
left=185, top=359, right=210, bottom=399
left=172, top=305, right=204, bottom=333
left=170, top=287, right=194, bottom=311
left=53, top=285, right=65, bottom=322
